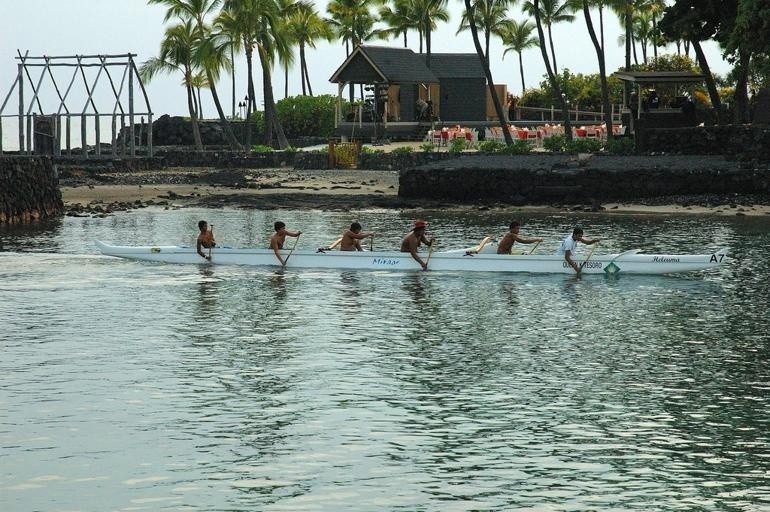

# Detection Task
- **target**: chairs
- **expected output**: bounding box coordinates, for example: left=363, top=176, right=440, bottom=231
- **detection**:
left=424, top=123, right=626, bottom=147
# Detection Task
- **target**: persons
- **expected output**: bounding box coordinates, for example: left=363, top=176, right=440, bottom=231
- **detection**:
left=197, top=220, right=232, bottom=260
left=416, top=96, right=429, bottom=121
left=629, top=92, right=639, bottom=106
left=340, top=222, right=383, bottom=251
left=401, top=219, right=435, bottom=268
left=497, top=221, right=543, bottom=254
left=557, top=228, right=600, bottom=272
left=649, top=91, right=661, bottom=109
left=268, top=221, right=302, bottom=266
left=507, top=94, right=517, bottom=121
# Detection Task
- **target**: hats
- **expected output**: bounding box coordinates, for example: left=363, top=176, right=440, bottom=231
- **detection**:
left=412, top=222, right=426, bottom=229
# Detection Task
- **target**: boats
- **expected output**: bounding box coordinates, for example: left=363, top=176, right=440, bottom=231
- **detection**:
left=90, top=239, right=730, bottom=276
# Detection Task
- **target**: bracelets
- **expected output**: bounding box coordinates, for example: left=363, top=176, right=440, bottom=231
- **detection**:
left=205, top=255, right=207, bottom=259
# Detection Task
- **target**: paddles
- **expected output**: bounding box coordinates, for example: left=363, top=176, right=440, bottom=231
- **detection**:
left=577, top=238, right=597, bottom=281
